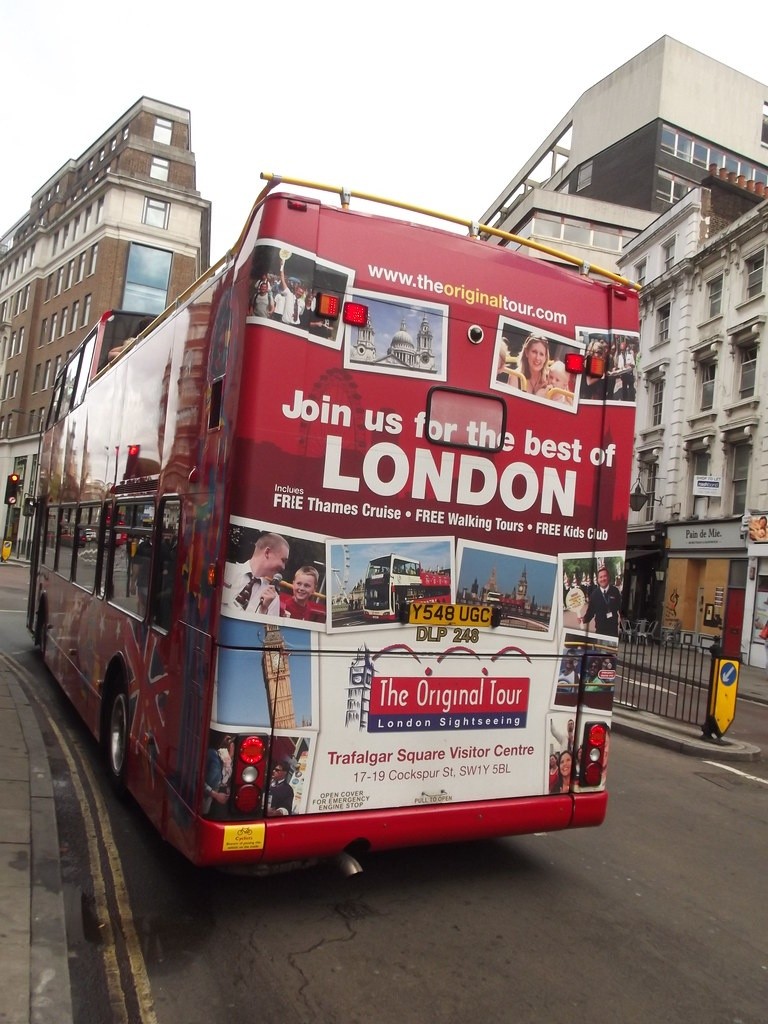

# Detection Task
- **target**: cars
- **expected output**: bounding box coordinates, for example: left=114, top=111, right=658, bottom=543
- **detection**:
left=485, top=590, right=500, bottom=605
left=38, top=507, right=167, bottom=552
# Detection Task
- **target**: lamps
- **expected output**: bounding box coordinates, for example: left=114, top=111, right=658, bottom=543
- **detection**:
left=655, top=571, right=665, bottom=581
left=629, top=464, right=649, bottom=511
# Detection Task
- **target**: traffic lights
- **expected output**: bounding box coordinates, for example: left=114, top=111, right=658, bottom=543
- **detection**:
left=4, top=472, right=17, bottom=505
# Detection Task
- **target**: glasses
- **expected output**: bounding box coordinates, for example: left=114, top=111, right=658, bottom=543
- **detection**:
left=524, top=332, right=549, bottom=344
left=588, top=664, right=600, bottom=670
left=273, top=767, right=283, bottom=772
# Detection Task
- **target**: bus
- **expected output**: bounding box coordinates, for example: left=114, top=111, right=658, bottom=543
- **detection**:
left=361, top=552, right=448, bottom=624
left=22, top=169, right=645, bottom=886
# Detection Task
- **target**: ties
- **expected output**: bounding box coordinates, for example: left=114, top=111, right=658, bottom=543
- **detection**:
left=293, top=298, right=299, bottom=322
left=234, top=572, right=261, bottom=611
left=567, top=737, right=571, bottom=751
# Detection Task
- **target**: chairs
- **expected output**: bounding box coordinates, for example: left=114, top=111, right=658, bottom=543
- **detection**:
left=620, top=617, right=681, bottom=648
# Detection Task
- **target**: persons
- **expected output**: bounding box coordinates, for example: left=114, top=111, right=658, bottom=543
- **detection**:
left=497, top=335, right=509, bottom=384
left=550, top=717, right=583, bottom=793
left=507, top=333, right=552, bottom=395
left=280, top=567, right=326, bottom=622
left=222, top=534, right=290, bottom=617
left=559, top=651, right=615, bottom=692
left=581, top=335, right=636, bottom=401
left=203, top=737, right=234, bottom=818
left=247, top=264, right=335, bottom=339
left=582, top=570, right=621, bottom=637
left=129, top=521, right=177, bottom=627
left=263, top=762, right=294, bottom=816
left=537, top=361, right=572, bottom=405
left=749, top=516, right=767, bottom=540
